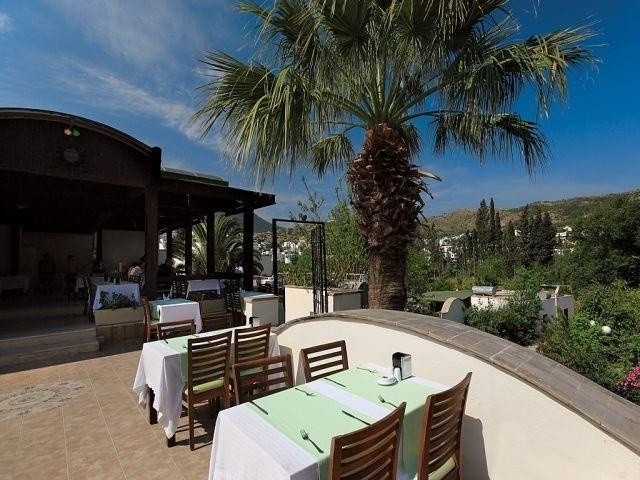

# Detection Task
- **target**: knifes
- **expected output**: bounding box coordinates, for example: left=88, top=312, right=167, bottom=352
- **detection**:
left=323, top=377, right=346, bottom=387
left=164, top=338, right=168, bottom=344
left=342, top=410, right=371, bottom=426
left=249, top=400, right=268, bottom=415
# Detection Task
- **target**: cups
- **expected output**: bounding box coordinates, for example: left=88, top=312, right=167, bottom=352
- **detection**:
left=379, top=375, right=395, bottom=383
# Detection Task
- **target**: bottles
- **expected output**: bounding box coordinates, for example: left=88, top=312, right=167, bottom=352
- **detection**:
left=393, top=368, right=401, bottom=382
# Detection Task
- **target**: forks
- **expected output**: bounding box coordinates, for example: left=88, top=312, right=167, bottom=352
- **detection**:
left=300, top=428, right=323, bottom=453
left=377, top=394, right=398, bottom=409
left=295, top=388, right=314, bottom=396
left=356, top=367, right=376, bottom=373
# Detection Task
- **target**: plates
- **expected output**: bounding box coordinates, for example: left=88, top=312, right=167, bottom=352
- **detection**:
left=377, top=380, right=396, bottom=386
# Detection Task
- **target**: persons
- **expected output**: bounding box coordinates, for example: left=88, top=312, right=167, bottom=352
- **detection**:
left=130, top=263, right=146, bottom=290
left=37, top=251, right=53, bottom=279
left=62, top=254, right=77, bottom=292
left=157, top=257, right=175, bottom=290
left=127, top=262, right=137, bottom=280
